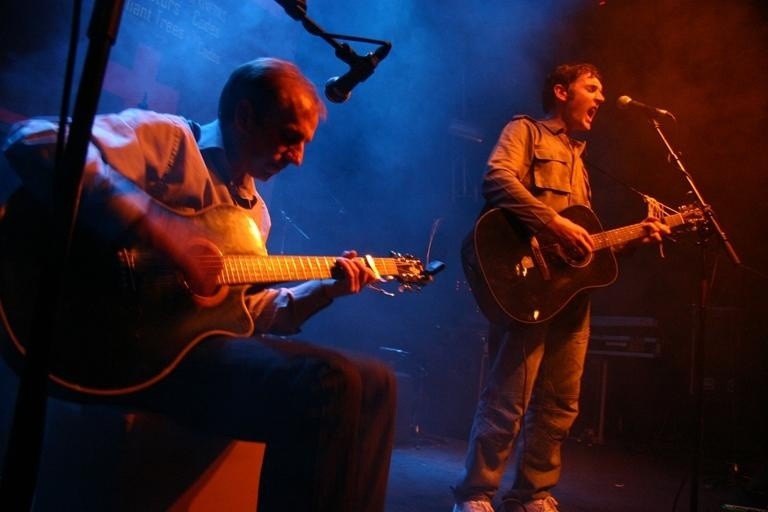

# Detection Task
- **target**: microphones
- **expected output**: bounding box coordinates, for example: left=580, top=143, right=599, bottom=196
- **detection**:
left=324, top=41, right=392, bottom=104
left=615, top=96, right=672, bottom=119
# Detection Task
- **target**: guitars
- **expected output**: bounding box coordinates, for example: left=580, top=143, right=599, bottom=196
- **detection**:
left=461, top=202, right=711, bottom=325
left=0, top=178, right=446, bottom=395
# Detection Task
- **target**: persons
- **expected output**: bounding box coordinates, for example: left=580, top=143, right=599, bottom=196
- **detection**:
left=451, top=61, right=672, bottom=512
left=0, top=56, right=397, bottom=512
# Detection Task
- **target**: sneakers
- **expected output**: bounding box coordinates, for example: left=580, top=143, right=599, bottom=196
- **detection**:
left=509, top=497, right=558, bottom=510
left=450, top=501, right=494, bottom=511
